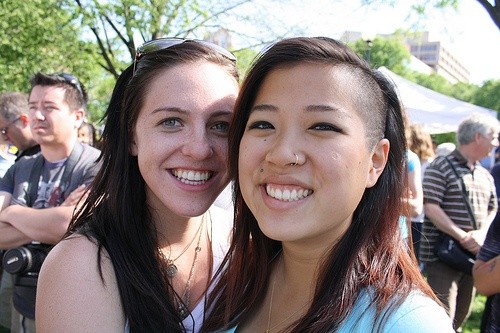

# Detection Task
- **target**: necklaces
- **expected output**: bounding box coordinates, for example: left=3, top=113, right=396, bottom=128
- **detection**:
left=165, top=214, right=205, bottom=323
left=265, top=251, right=314, bottom=333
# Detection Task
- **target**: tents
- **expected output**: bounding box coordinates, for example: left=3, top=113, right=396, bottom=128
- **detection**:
left=376, top=65, right=498, bottom=135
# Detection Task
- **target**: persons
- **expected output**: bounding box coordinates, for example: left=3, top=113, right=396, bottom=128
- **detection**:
left=199, top=37, right=456, bottom=333
left=398, top=114, right=500, bottom=333
left=0, top=73, right=112, bottom=333
left=34, top=41, right=252, bottom=333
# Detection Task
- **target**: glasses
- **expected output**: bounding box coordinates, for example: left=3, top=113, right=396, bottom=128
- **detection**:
left=63, top=74, right=84, bottom=97
left=134, top=37, right=237, bottom=71
left=1, top=116, right=20, bottom=135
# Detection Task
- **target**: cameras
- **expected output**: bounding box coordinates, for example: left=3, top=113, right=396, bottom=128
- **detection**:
left=1, top=241, right=55, bottom=276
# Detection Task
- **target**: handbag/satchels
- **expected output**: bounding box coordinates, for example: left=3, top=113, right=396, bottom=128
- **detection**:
left=433, top=234, right=476, bottom=276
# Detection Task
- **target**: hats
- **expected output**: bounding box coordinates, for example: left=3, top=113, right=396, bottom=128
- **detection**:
left=435, top=142, right=456, bottom=156
